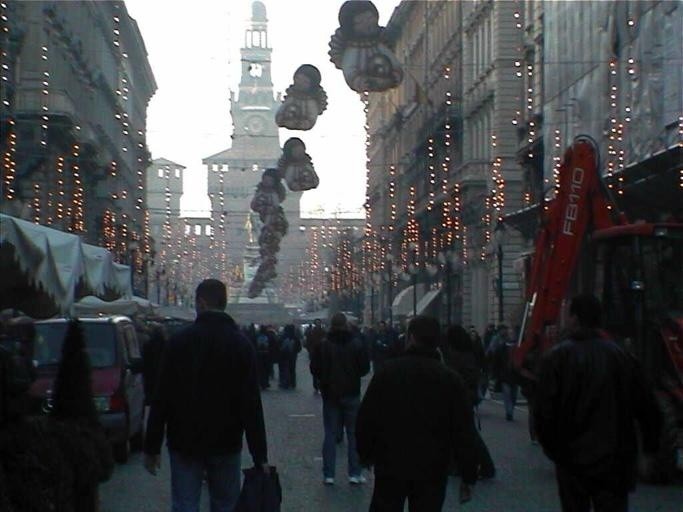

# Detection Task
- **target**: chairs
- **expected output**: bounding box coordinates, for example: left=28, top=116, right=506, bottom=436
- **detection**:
left=321, top=476, right=336, bottom=485
left=349, top=474, right=368, bottom=484
left=459, top=482, right=472, bottom=505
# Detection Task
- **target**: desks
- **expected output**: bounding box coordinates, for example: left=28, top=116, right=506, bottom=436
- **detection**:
left=366, top=224, right=507, bottom=331
left=128, top=238, right=195, bottom=308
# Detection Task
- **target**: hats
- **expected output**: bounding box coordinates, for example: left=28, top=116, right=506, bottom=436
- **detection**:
left=27, top=316, right=148, bottom=462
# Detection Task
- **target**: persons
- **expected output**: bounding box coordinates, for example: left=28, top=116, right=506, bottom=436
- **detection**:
left=352, top=316, right=481, bottom=511
left=234, top=313, right=521, bottom=441
left=529, top=295, right=661, bottom=511
left=308, top=312, right=371, bottom=486
left=137, top=277, right=271, bottom=512
left=513, top=330, right=550, bottom=446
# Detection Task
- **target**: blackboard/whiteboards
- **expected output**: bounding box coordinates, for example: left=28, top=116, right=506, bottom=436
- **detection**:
left=505, top=145, right=680, bottom=484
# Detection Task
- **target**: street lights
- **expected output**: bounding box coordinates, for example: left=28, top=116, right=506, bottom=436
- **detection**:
left=231, top=464, right=283, bottom=511
